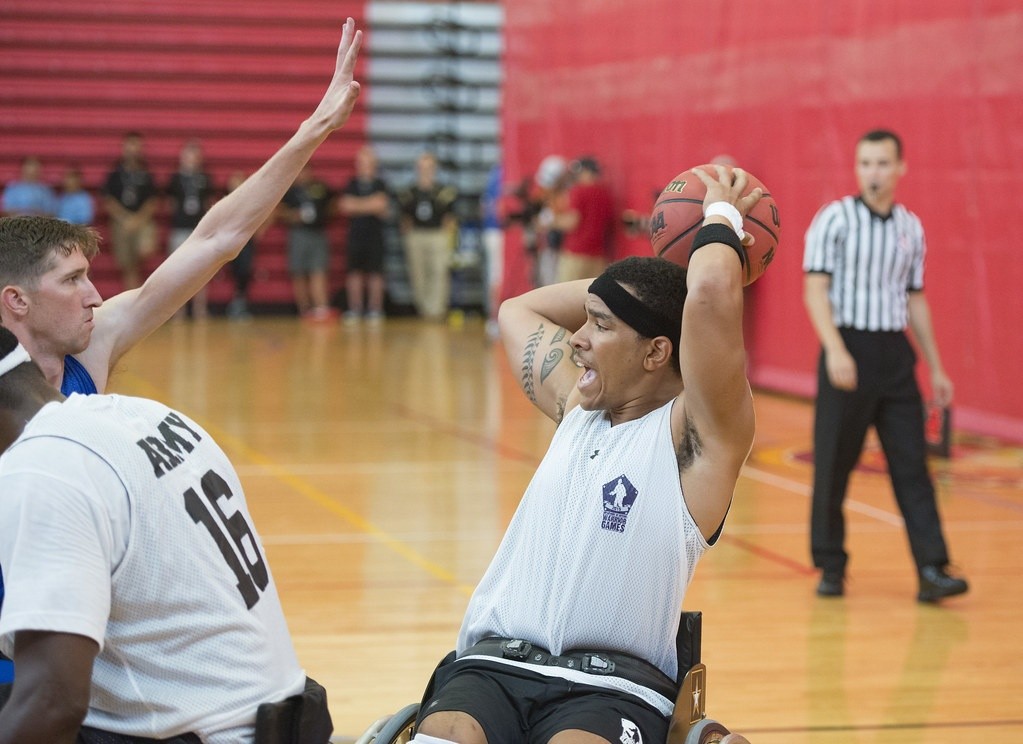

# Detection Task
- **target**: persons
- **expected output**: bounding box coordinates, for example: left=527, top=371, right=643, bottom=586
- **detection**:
left=2, top=11, right=363, bottom=695
left=398, top=163, right=761, bottom=744
left=802, top=125, right=977, bottom=603
left=0, top=325, right=328, bottom=744
left=1, top=113, right=625, bottom=338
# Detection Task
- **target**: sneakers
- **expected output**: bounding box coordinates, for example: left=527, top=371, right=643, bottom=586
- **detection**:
left=919, top=567, right=966, bottom=600
left=818, top=570, right=843, bottom=595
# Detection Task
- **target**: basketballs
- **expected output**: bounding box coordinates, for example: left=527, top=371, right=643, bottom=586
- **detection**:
left=650, top=164, right=781, bottom=289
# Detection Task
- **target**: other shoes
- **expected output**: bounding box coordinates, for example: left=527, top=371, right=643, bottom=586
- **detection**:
left=304, top=310, right=386, bottom=325
